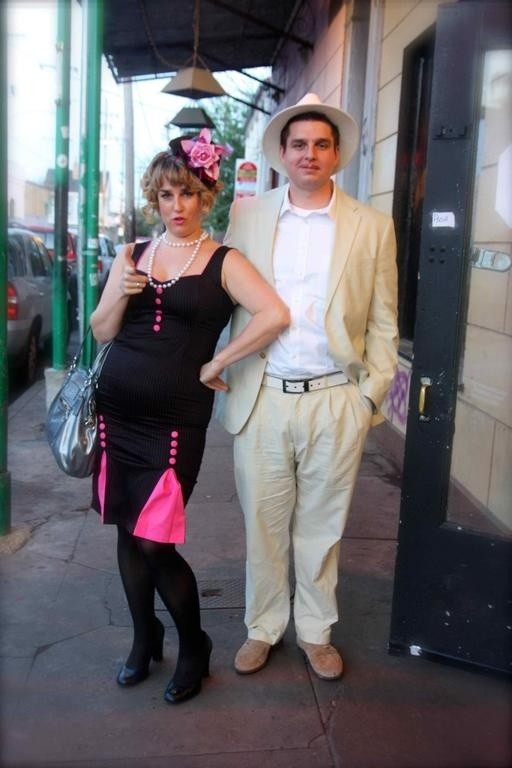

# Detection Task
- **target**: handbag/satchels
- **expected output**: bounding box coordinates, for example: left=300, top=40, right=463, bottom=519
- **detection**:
left=45, top=357, right=103, bottom=479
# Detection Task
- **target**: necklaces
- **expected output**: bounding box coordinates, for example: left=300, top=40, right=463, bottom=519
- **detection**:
left=146, top=228, right=210, bottom=289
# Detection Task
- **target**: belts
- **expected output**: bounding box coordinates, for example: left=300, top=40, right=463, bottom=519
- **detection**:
left=256, top=359, right=351, bottom=393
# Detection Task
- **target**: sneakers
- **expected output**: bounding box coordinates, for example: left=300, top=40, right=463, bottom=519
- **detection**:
left=232, top=633, right=286, bottom=675
left=291, top=625, right=346, bottom=682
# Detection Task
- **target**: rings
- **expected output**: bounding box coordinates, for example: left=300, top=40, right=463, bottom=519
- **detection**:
left=136, top=281, right=140, bottom=288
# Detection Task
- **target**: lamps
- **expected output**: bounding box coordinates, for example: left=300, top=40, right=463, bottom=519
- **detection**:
left=161, top=1, right=283, bottom=129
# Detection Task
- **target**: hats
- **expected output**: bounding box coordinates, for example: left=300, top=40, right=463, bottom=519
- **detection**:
left=258, top=89, right=360, bottom=182
left=169, top=130, right=225, bottom=193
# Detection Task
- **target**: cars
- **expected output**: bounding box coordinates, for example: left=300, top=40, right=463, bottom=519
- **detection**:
left=6, top=224, right=117, bottom=388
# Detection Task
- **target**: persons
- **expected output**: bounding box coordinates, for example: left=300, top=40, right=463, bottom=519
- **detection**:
left=86, top=134, right=290, bottom=703
left=222, top=90, right=400, bottom=680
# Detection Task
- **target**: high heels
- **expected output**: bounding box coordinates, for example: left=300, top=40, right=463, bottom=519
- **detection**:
left=113, top=622, right=165, bottom=689
left=165, top=630, right=212, bottom=708
